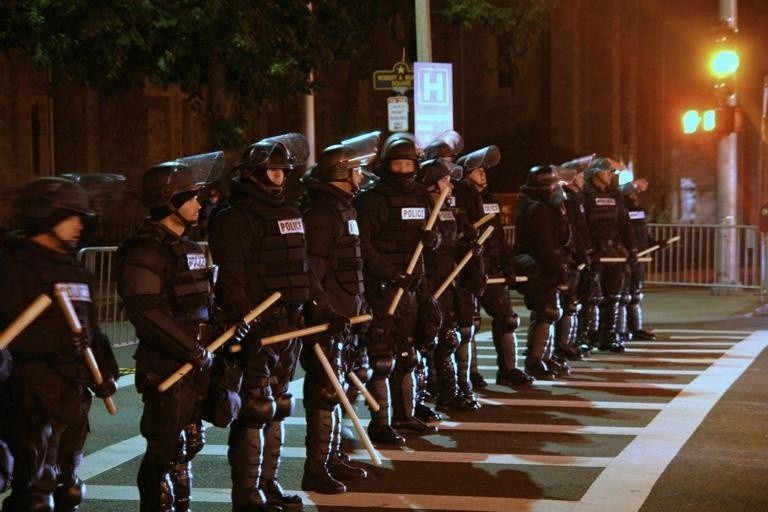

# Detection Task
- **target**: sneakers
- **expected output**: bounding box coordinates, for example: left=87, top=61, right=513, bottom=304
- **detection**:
left=299, top=468, right=347, bottom=496
left=366, top=372, right=488, bottom=446
left=325, top=457, right=369, bottom=481
left=525, top=327, right=657, bottom=381
left=230, top=480, right=303, bottom=512
left=494, top=367, right=536, bottom=387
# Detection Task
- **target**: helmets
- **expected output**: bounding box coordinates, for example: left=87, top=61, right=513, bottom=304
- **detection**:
left=140, top=149, right=226, bottom=210
left=525, top=151, right=642, bottom=192
left=236, top=130, right=498, bottom=190
left=16, top=170, right=127, bottom=223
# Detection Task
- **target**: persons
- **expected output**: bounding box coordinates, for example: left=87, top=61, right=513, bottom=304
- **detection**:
left=759, top=205, right=768, bottom=284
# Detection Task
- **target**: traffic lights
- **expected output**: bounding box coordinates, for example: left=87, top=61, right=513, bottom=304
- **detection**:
left=706, top=27, right=738, bottom=98
left=674, top=108, right=726, bottom=137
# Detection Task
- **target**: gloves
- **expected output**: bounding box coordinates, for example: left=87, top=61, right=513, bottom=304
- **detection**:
left=388, top=270, right=414, bottom=290
left=466, top=240, right=484, bottom=258
left=658, top=240, right=668, bottom=249
left=580, top=252, right=638, bottom=271
left=505, top=271, right=517, bottom=286
left=418, top=229, right=442, bottom=253
left=327, top=312, right=351, bottom=338
left=59, top=325, right=92, bottom=354
left=187, top=312, right=263, bottom=377
left=92, top=371, right=118, bottom=399
